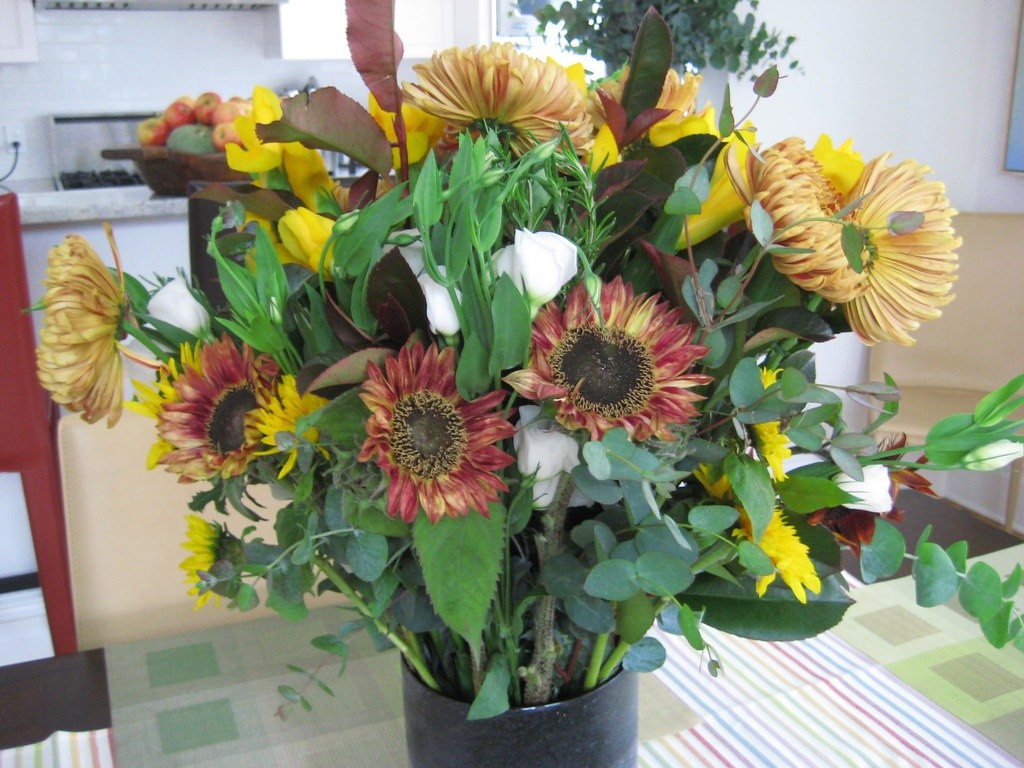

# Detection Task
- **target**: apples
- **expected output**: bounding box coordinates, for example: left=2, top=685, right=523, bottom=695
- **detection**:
left=136, top=93, right=254, bottom=151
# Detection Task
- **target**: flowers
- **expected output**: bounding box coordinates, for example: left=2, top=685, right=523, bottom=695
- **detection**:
left=16, top=1, right=1024, bottom=720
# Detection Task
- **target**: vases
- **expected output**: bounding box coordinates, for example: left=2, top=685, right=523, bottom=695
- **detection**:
left=396, top=652, right=642, bottom=768
